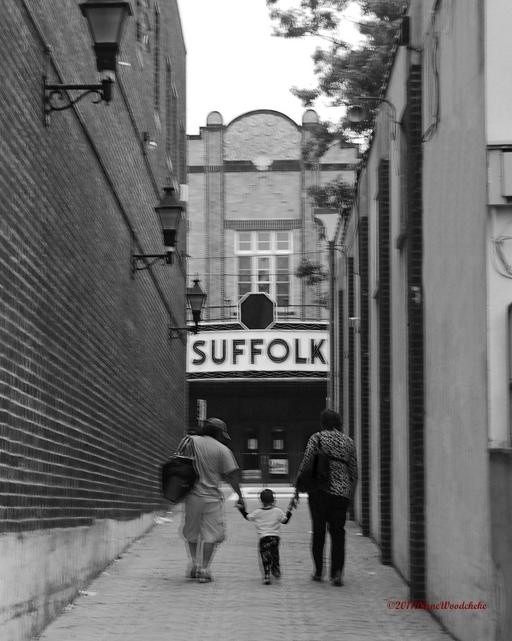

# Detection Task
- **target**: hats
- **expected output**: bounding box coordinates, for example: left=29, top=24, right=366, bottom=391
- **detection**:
left=207, top=418, right=232, bottom=440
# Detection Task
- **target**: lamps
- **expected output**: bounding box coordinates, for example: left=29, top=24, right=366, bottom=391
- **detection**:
left=344, top=92, right=393, bottom=123
left=131, top=181, right=188, bottom=278
left=40, top=1, right=133, bottom=132
left=166, top=278, right=205, bottom=339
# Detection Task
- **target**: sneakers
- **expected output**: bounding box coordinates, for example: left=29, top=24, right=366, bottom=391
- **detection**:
left=191, top=568, right=212, bottom=583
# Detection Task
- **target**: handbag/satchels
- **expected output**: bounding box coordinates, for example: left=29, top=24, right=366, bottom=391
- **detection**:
left=297, top=432, right=329, bottom=491
left=162, top=436, right=197, bottom=503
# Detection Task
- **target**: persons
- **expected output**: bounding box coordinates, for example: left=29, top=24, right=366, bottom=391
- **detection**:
left=161, top=409, right=358, bottom=587
